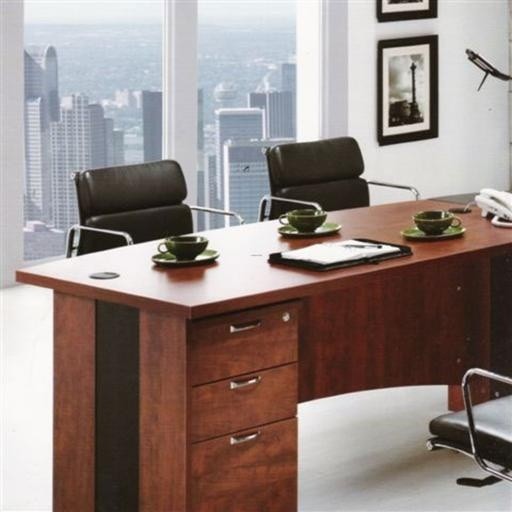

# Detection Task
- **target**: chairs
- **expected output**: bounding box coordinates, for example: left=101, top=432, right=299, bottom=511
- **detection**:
left=258, top=136, right=420, bottom=222
left=425, top=367, right=512, bottom=487
left=65, top=159, right=245, bottom=258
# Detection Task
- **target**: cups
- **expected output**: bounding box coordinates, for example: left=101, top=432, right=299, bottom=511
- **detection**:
left=278, top=209, right=327, bottom=232
left=158, top=236, right=208, bottom=260
left=413, top=209, right=462, bottom=235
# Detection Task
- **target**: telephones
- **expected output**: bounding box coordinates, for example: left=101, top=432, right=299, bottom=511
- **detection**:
left=475, top=189, right=512, bottom=221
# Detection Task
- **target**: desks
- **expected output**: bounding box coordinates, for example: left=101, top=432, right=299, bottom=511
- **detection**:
left=15, top=190, right=512, bottom=512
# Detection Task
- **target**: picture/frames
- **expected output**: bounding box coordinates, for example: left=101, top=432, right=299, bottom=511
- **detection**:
left=377, top=0, right=437, bottom=22
left=376, top=34, right=438, bottom=146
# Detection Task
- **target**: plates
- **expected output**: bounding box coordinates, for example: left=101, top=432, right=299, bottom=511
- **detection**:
left=400, top=224, right=466, bottom=240
left=278, top=223, right=342, bottom=237
left=151, top=250, right=219, bottom=267
